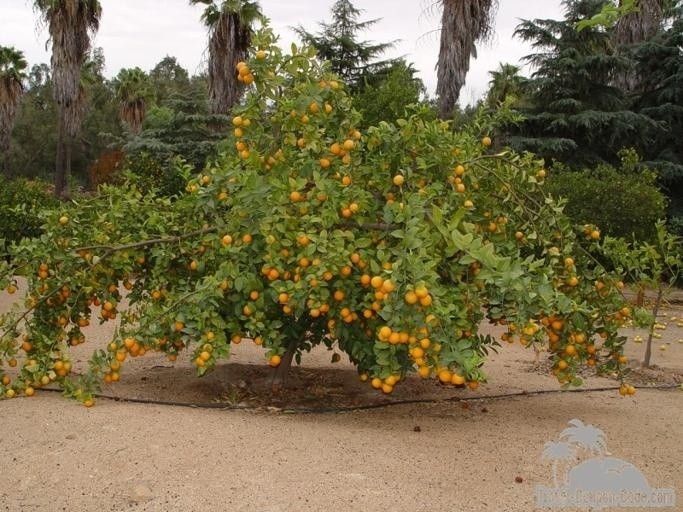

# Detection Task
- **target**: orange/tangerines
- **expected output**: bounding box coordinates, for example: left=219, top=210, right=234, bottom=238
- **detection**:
left=634, top=313, right=683, bottom=350
left=0, top=49, right=636, bottom=408
